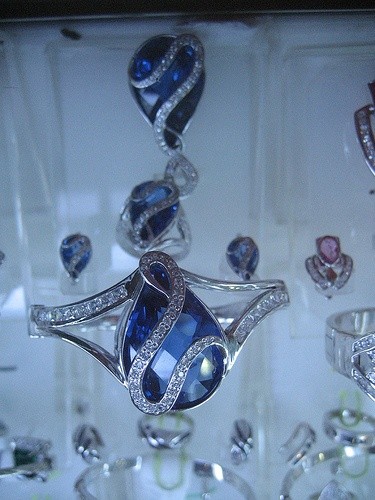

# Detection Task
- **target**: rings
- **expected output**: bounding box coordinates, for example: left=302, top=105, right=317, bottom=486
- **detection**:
left=0, top=32, right=375, bottom=500
